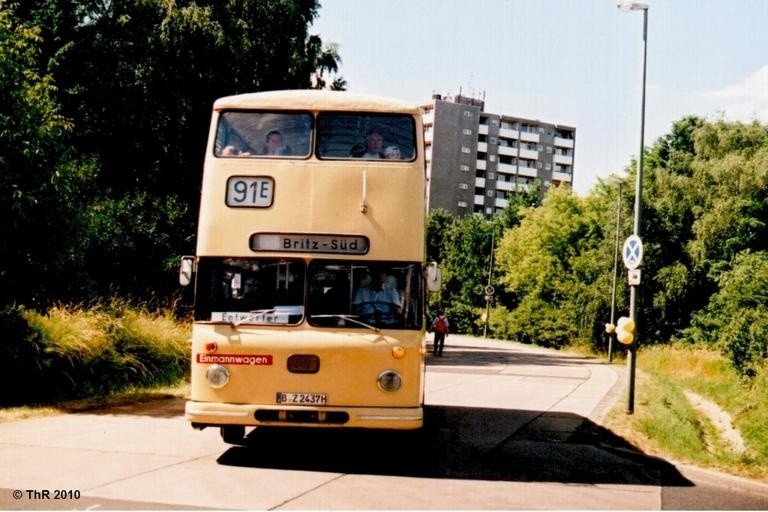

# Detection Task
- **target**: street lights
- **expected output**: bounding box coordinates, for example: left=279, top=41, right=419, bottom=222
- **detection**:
left=483, top=219, right=504, bottom=337
left=606, top=1, right=648, bottom=416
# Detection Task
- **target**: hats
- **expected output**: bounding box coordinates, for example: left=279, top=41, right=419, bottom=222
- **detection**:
left=384, top=145, right=400, bottom=156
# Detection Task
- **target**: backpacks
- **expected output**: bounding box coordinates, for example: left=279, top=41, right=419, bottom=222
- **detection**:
left=435, top=316, right=446, bottom=335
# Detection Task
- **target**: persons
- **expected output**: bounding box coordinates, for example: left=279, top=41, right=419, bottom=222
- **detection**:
left=383, top=145, right=400, bottom=159
left=222, top=144, right=252, bottom=156
left=260, top=130, right=282, bottom=154
left=347, top=127, right=385, bottom=159
left=274, top=144, right=293, bottom=156
left=347, top=145, right=364, bottom=158
left=349, top=265, right=402, bottom=324
left=427, top=309, right=449, bottom=357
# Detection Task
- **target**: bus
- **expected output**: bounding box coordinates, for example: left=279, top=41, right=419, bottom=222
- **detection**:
left=179, top=88, right=442, bottom=441
